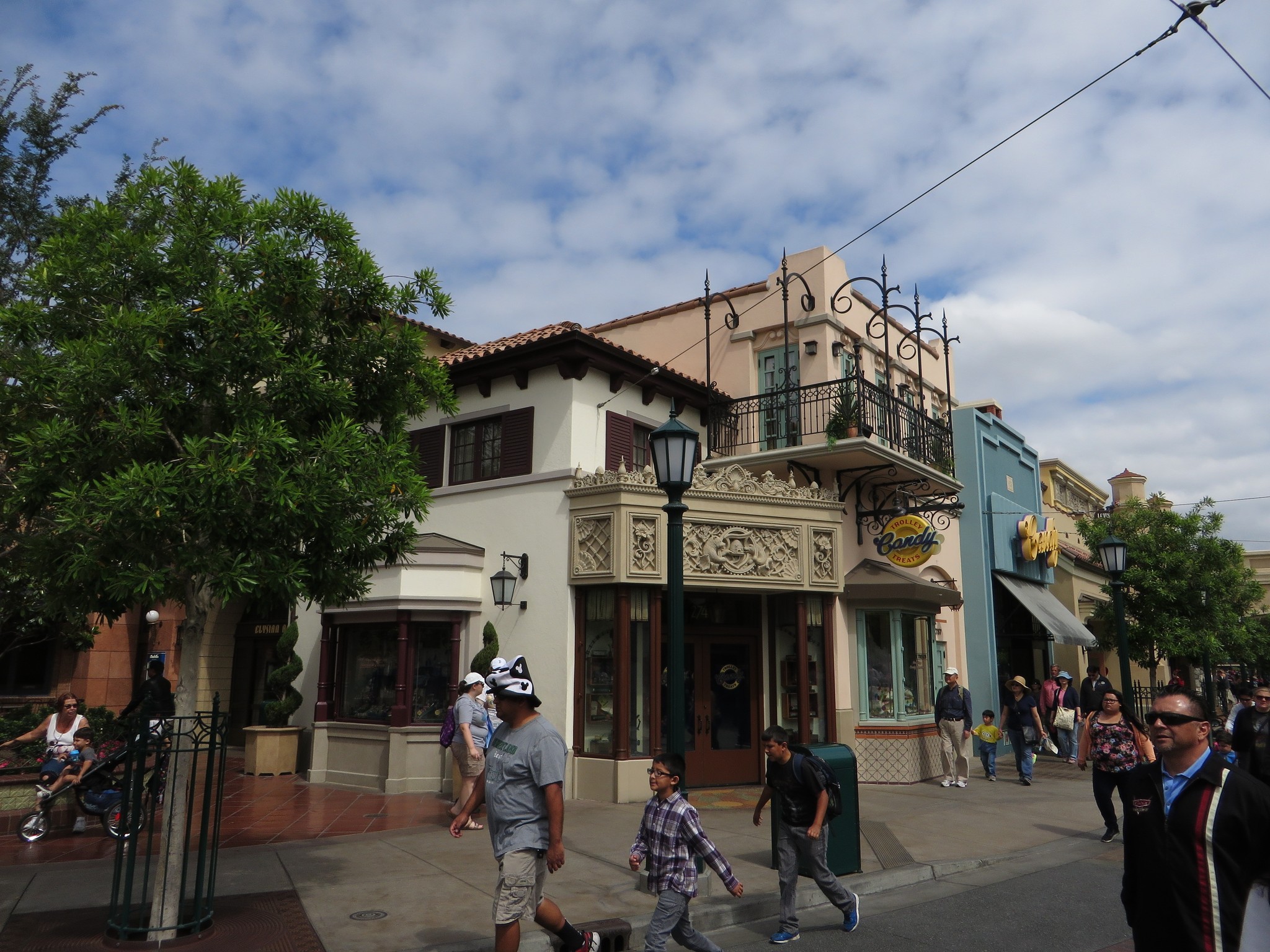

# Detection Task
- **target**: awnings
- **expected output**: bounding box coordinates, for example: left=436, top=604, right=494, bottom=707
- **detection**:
left=995, top=575, right=1100, bottom=648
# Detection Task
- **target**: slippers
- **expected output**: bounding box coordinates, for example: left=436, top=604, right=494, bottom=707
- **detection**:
left=446, top=810, right=483, bottom=830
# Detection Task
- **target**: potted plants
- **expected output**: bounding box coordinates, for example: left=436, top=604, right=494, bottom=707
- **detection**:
left=930, top=440, right=958, bottom=476
left=242, top=620, right=305, bottom=777
left=826, top=371, right=868, bottom=438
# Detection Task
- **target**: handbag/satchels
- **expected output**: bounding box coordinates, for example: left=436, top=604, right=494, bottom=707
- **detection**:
left=1021, top=726, right=1039, bottom=744
left=1053, top=706, right=1075, bottom=730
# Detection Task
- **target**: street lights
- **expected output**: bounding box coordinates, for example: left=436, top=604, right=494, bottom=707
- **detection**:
left=1194, top=590, right=1217, bottom=713
left=647, top=392, right=709, bottom=872
left=1095, top=526, right=1139, bottom=715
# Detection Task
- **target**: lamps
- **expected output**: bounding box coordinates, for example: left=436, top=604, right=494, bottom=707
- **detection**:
left=930, top=577, right=964, bottom=612
left=146, top=610, right=162, bottom=646
left=489, top=550, right=528, bottom=609
left=833, top=340, right=846, bottom=356
left=890, top=485, right=907, bottom=517
left=803, top=339, right=818, bottom=354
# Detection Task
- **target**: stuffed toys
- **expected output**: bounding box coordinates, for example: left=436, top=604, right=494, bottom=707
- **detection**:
left=866, top=643, right=919, bottom=718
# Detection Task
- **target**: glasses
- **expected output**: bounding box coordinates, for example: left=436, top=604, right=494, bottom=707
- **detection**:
left=647, top=768, right=673, bottom=778
left=63, top=704, right=78, bottom=709
left=1238, top=695, right=1270, bottom=700
left=1088, top=674, right=1095, bottom=676
left=1144, top=713, right=1205, bottom=727
left=479, top=682, right=484, bottom=686
left=1102, top=698, right=1118, bottom=704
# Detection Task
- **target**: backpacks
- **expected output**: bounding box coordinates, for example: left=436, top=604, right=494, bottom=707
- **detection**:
left=439, top=697, right=468, bottom=749
left=789, top=745, right=842, bottom=820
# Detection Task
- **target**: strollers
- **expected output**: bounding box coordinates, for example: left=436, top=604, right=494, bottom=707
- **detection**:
left=16, top=713, right=162, bottom=844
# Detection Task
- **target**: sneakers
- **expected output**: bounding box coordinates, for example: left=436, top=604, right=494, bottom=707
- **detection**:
left=938, top=755, right=1075, bottom=792
left=1101, top=828, right=1120, bottom=842
left=575, top=929, right=600, bottom=952
left=35, top=783, right=52, bottom=798
left=23, top=817, right=45, bottom=831
left=73, top=819, right=86, bottom=833
left=769, top=893, right=860, bottom=944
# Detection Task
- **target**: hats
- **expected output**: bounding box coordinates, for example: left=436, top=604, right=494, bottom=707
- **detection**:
left=1004, top=670, right=1073, bottom=693
left=1043, top=738, right=1058, bottom=754
left=463, top=655, right=541, bottom=709
left=943, top=667, right=958, bottom=675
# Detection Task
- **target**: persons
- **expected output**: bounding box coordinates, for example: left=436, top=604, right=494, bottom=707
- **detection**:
left=751, top=723, right=861, bottom=944
left=448, top=656, right=602, bottom=952
left=0, top=693, right=90, bottom=832
left=35, top=726, right=96, bottom=799
left=971, top=709, right=1003, bottom=782
left=1169, top=665, right=1270, bottom=690
left=1232, top=688, right=1270, bottom=788
left=998, top=663, right=1112, bottom=786
left=627, top=752, right=743, bottom=952
left=1225, top=687, right=1258, bottom=735
left=1077, top=688, right=1157, bottom=844
left=1121, top=684, right=1269, bottom=952
left=119, top=660, right=173, bottom=719
left=933, top=669, right=973, bottom=787
left=1216, top=732, right=1240, bottom=768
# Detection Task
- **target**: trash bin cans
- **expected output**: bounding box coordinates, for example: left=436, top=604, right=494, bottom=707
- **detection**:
left=767, top=743, right=863, bottom=877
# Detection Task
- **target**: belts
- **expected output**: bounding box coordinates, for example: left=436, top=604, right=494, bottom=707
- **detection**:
left=941, top=716, right=964, bottom=722
